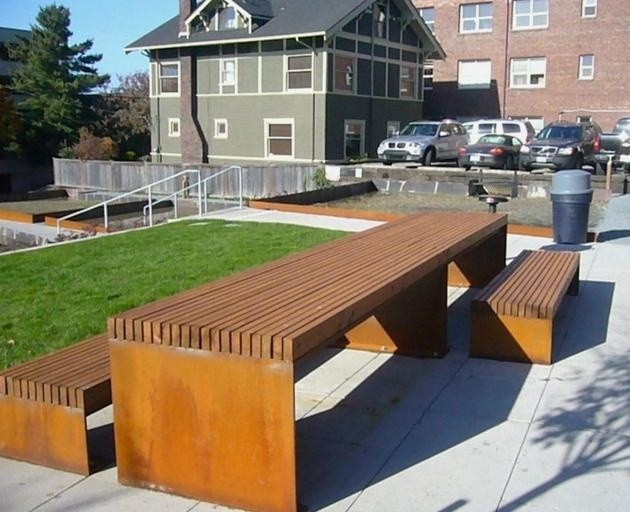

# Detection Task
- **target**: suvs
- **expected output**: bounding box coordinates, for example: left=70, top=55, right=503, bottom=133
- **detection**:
left=345, top=130, right=361, bottom=156
left=377, top=118, right=471, bottom=167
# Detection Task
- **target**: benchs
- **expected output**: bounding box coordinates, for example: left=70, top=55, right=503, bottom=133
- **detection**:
left=465, top=245, right=584, bottom=367
left=1, top=332, right=112, bottom=480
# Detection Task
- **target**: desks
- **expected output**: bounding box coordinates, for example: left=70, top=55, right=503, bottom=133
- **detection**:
left=104, top=206, right=511, bottom=512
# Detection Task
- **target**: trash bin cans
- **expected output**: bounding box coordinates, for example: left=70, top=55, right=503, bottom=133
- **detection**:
left=550, top=169, right=593, bottom=244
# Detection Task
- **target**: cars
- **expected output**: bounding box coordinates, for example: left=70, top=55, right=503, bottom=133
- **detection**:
left=458, top=117, right=630, bottom=176
left=389, top=128, right=400, bottom=137
